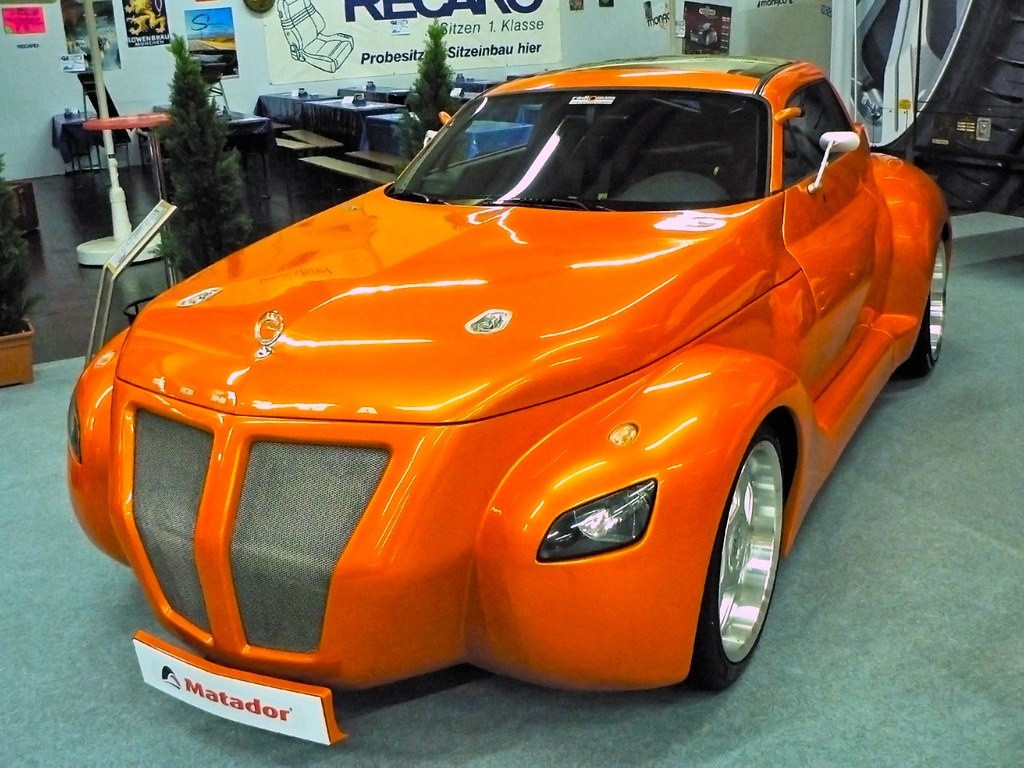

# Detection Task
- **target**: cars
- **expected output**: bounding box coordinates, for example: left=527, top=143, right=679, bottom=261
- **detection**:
left=62, top=55, right=956, bottom=749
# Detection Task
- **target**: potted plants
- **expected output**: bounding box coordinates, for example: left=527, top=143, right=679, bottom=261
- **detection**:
left=0, top=149, right=44, bottom=387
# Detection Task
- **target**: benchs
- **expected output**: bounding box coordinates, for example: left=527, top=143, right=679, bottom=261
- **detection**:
left=266, top=120, right=414, bottom=199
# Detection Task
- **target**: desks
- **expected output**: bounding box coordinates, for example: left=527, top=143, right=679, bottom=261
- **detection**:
left=453, top=79, right=501, bottom=93
left=447, top=120, right=534, bottom=165
left=303, top=98, right=407, bottom=152
left=365, top=114, right=420, bottom=156
left=253, top=92, right=342, bottom=127
left=454, top=92, right=480, bottom=117
left=51, top=112, right=109, bottom=195
left=154, top=104, right=281, bottom=200
left=337, top=85, right=410, bottom=105
